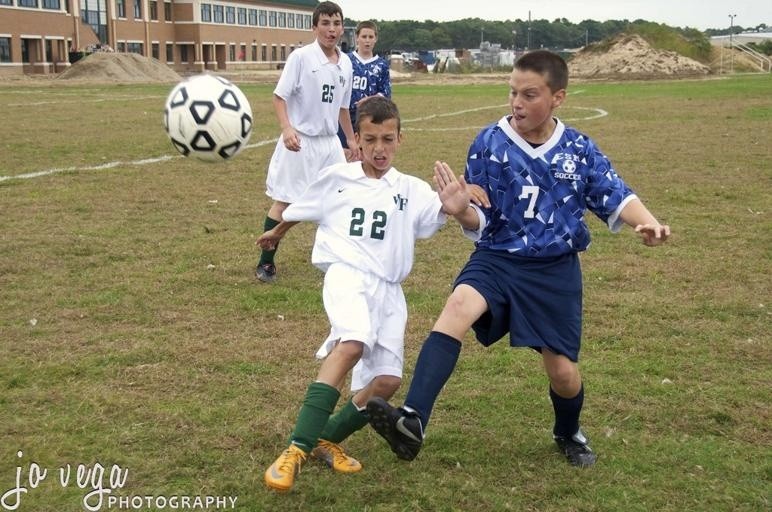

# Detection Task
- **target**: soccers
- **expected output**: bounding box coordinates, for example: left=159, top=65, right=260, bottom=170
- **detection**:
left=164, top=77, right=252, bottom=162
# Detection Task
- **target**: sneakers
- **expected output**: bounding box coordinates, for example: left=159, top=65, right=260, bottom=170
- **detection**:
left=312, top=438, right=362, bottom=472
left=553, top=426, right=593, bottom=467
left=265, top=444, right=309, bottom=490
left=257, top=265, right=276, bottom=283
left=366, top=398, right=425, bottom=461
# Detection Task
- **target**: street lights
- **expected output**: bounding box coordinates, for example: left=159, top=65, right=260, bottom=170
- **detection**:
left=729, top=13, right=736, bottom=49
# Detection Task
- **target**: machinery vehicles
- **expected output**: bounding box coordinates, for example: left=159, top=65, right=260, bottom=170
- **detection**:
left=68, top=43, right=114, bottom=65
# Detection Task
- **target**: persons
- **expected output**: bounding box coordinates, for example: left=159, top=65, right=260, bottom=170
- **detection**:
left=253, top=0, right=364, bottom=287
left=249, top=95, right=494, bottom=494
left=335, top=18, right=393, bottom=152
left=363, top=49, right=674, bottom=474
